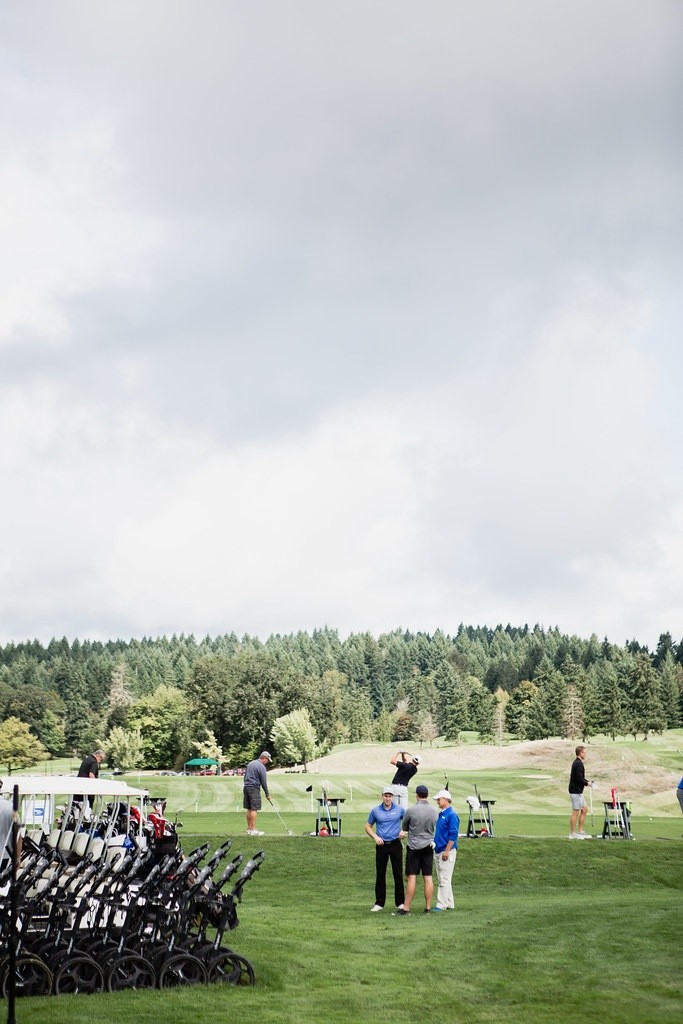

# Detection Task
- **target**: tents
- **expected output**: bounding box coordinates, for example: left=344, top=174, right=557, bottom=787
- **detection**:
left=184, top=759, right=221, bottom=776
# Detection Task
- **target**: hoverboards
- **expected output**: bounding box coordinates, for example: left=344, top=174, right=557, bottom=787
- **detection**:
left=0, top=840, right=268, bottom=997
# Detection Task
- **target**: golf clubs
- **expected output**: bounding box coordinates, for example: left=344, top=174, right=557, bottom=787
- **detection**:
left=55, top=800, right=79, bottom=816
left=268, top=798, right=294, bottom=836
left=589, top=781, right=596, bottom=828
left=611, top=788, right=629, bottom=840
left=473, top=783, right=492, bottom=838
left=403, top=751, right=447, bottom=779
left=319, top=784, right=334, bottom=837
left=173, top=808, right=183, bottom=830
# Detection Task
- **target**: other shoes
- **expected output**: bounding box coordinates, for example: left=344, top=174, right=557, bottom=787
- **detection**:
left=569, top=833, right=584, bottom=839
left=578, top=833, right=592, bottom=838
left=247, top=830, right=264, bottom=835
left=424, top=909, right=430, bottom=915
left=432, top=907, right=450, bottom=911
left=397, top=909, right=408, bottom=915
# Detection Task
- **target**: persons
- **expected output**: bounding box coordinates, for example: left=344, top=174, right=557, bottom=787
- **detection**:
left=676, top=777, right=683, bottom=838
left=390, top=751, right=423, bottom=834
left=391, top=785, right=439, bottom=915
left=568, top=746, right=592, bottom=840
left=364, top=787, right=406, bottom=911
left=73, top=749, right=106, bottom=811
left=433, top=790, right=460, bottom=911
left=0, top=779, right=26, bottom=872
left=243, top=751, right=272, bottom=836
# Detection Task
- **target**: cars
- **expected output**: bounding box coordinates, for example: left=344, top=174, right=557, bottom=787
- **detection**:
left=100, top=771, right=124, bottom=775
left=153, top=770, right=238, bottom=776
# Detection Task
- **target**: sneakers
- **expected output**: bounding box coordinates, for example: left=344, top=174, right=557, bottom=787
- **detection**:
left=371, top=905, right=383, bottom=912
left=396, top=904, right=404, bottom=909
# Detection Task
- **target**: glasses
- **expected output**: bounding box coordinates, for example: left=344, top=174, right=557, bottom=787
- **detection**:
left=99, top=756, right=103, bottom=762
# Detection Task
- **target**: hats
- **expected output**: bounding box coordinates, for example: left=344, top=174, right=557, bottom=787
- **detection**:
left=414, top=756, right=421, bottom=763
left=382, top=787, right=393, bottom=795
left=433, top=790, right=451, bottom=800
left=261, top=751, right=273, bottom=762
